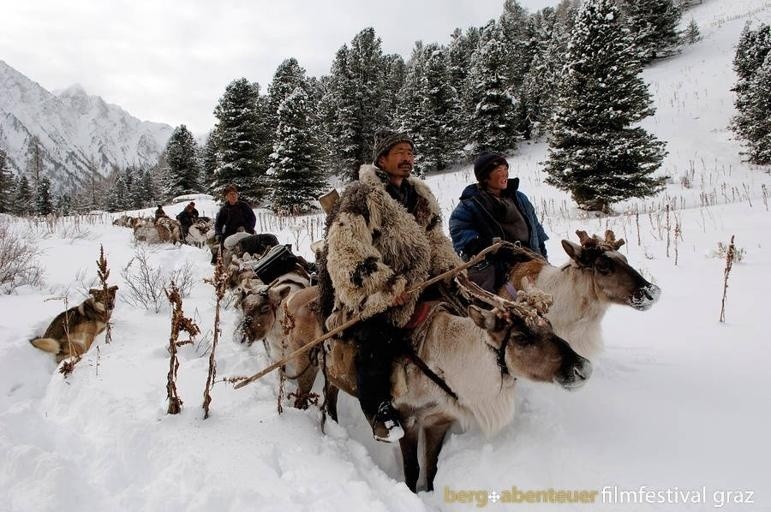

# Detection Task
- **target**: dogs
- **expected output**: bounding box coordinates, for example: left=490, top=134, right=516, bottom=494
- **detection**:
left=28, top=285, right=119, bottom=365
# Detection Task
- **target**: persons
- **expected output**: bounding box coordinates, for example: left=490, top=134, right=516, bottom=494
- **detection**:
left=155, top=205, right=165, bottom=221
left=450, top=153, right=549, bottom=295
left=190, top=202, right=199, bottom=218
left=214, top=185, right=256, bottom=259
left=176, top=205, right=194, bottom=237
left=324, top=125, right=469, bottom=440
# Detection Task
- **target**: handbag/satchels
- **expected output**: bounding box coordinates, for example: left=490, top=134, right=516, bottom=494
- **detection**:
left=252, top=243, right=298, bottom=285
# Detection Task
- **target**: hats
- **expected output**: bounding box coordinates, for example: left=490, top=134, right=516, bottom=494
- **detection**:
left=373, top=128, right=414, bottom=168
left=474, top=150, right=508, bottom=181
left=223, top=185, right=238, bottom=197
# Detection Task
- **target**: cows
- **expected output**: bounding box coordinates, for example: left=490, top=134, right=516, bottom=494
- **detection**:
left=443, top=229, right=663, bottom=363
left=317, top=271, right=594, bottom=494
left=112, top=215, right=218, bottom=249
left=221, top=247, right=321, bottom=412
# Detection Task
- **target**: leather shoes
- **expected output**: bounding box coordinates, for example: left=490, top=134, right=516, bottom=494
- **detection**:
left=368, top=401, right=406, bottom=443
